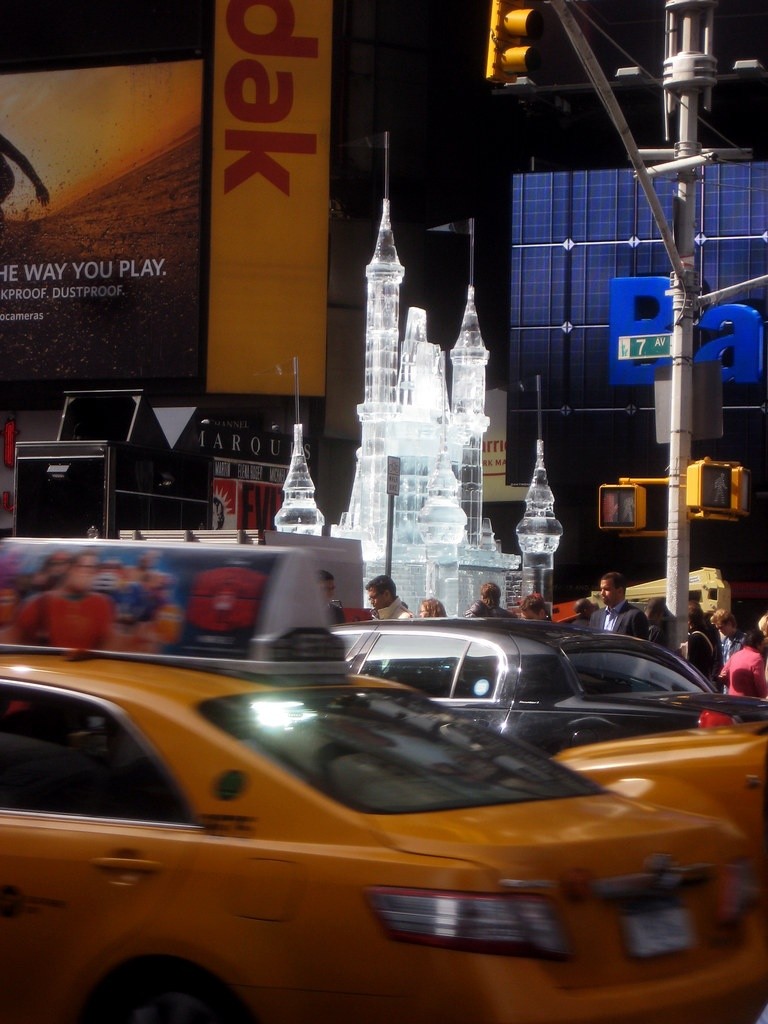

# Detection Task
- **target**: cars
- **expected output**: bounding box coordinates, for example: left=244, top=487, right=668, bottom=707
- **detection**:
left=550, top=721, right=768, bottom=866
left=1, top=533, right=768, bottom=1023
left=446, top=685, right=768, bottom=755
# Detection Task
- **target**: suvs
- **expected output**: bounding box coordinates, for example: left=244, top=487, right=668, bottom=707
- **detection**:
left=326, top=610, right=717, bottom=702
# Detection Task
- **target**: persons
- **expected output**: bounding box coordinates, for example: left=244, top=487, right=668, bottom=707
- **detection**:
left=0, top=550, right=180, bottom=654
left=316, top=571, right=768, bottom=701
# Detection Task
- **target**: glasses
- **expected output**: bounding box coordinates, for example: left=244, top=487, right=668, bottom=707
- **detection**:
left=369, top=593, right=382, bottom=600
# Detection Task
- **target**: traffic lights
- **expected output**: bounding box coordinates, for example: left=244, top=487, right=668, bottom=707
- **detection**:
left=483, top=0, right=546, bottom=84
left=730, top=466, right=752, bottom=518
left=598, top=483, right=647, bottom=532
left=685, top=460, right=733, bottom=515
